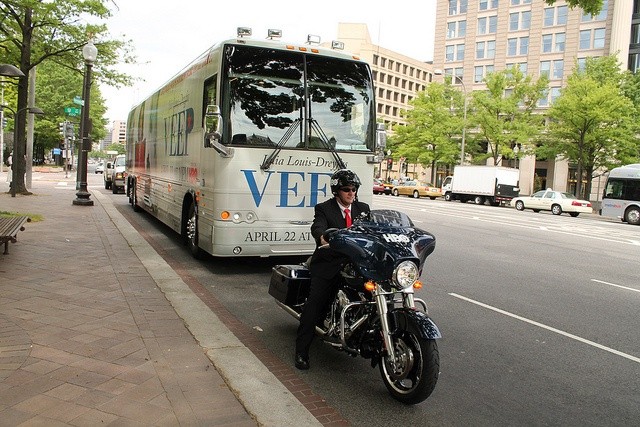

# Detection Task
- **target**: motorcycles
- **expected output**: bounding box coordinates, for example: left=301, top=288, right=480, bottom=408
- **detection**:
left=268, top=210, right=443, bottom=406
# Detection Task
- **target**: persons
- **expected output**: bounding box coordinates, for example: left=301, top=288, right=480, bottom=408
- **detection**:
left=295, top=169, right=370, bottom=370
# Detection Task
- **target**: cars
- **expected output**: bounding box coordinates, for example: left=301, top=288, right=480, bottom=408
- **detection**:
left=379, top=180, right=395, bottom=196
left=510, top=189, right=593, bottom=216
left=392, top=180, right=442, bottom=200
left=373, top=182, right=384, bottom=195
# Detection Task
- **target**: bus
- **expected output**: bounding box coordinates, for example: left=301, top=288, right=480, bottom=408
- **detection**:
left=125, top=37, right=376, bottom=261
left=600, top=164, right=640, bottom=226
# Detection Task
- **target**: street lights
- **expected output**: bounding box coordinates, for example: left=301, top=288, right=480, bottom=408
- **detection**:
left=434, top=68, right=468, bottom=168
left=0, top=104, right=44, bottom=197
left=0, top=64, right=25, bottom=78
left=70, top=37, right=97, bottom=207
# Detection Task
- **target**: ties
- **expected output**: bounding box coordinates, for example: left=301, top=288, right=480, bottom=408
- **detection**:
left=344, top=209, right=352, bottom=227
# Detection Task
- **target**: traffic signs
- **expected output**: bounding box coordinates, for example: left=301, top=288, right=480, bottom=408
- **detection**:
left=64, top=107, right=80, bottom=115
left=73, top=97, right=85, bottom=106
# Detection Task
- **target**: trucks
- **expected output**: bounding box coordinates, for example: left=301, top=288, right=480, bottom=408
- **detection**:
left=442, top=165, right=520, bottom=207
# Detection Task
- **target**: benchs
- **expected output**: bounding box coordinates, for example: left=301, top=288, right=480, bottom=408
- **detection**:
left=0, top=213, right=31, bottom=254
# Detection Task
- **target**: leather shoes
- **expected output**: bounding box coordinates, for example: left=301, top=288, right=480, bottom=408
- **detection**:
left=295, top=352, right=310, bottom=370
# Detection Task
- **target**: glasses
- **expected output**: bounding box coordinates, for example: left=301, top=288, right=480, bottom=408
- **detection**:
left=338, top=188, right=357, bottom=192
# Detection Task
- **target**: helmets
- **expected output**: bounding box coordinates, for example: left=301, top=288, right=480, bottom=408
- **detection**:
left=331, top=169, right=361, bottom=203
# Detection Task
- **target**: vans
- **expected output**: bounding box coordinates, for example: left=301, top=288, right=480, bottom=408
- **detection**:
left=111, top=155, right=126, bottom=194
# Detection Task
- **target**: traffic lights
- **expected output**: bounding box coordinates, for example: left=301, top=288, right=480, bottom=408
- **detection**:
left=387, top=158, right=392, bottom=168
